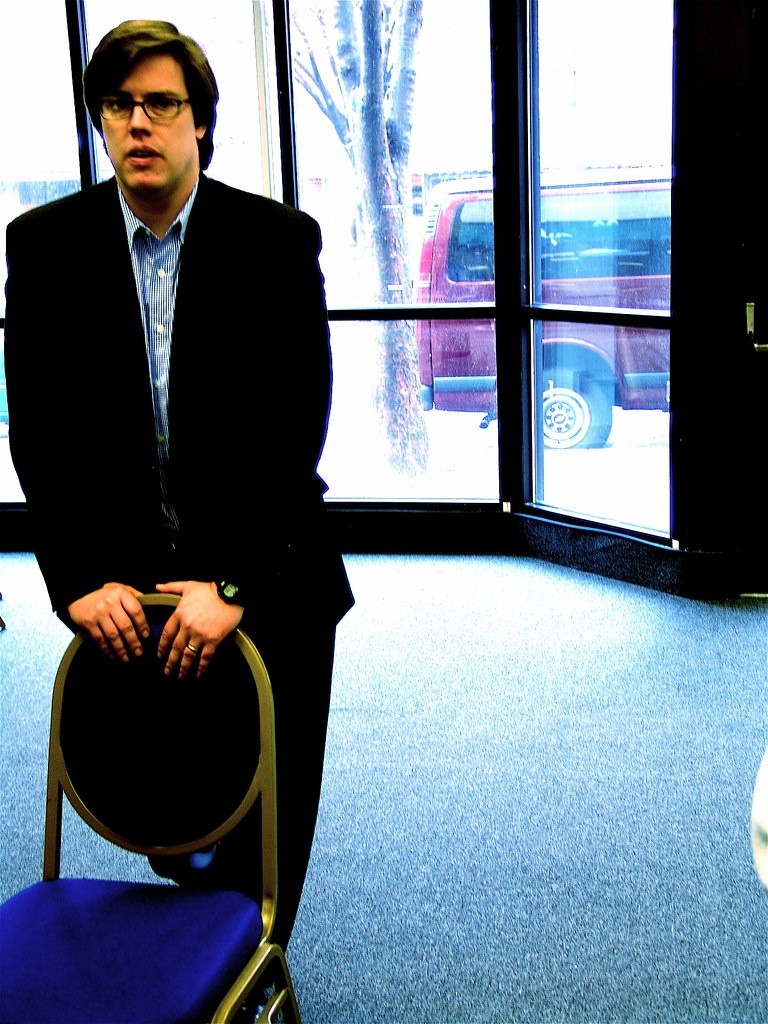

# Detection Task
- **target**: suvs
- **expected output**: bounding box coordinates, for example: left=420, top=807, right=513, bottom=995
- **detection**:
left=416, top=165, right=670, bottom=450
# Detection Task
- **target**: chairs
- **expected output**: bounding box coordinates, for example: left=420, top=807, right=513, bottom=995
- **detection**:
left=0, top=593, right=303, bottom=1024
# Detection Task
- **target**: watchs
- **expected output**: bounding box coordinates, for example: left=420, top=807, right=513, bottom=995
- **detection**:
left=213, top=575, right=249, bottom=608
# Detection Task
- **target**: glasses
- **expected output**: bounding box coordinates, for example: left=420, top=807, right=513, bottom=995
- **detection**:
left=98, top=96, right=190, bottom=120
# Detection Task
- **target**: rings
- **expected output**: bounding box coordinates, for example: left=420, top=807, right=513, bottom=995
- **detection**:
left=186, top=644, right=198, bottom=652
left=0, top=20, right=356, bottom=1024
